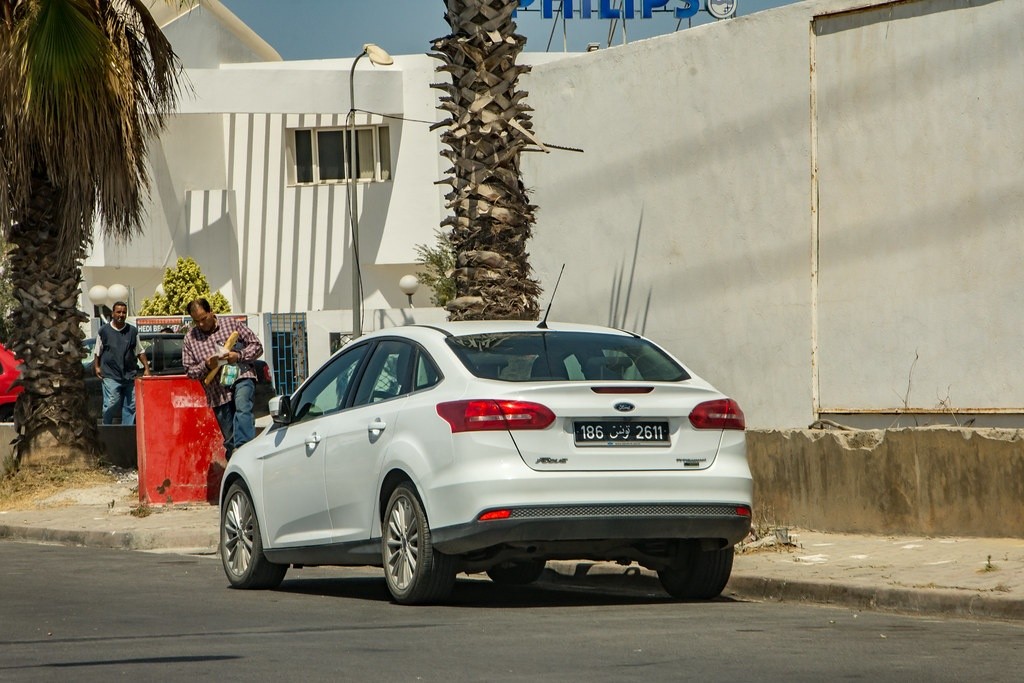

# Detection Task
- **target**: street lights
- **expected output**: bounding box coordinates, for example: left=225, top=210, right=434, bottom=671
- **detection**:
left=350, top=44, right=393, bottom=342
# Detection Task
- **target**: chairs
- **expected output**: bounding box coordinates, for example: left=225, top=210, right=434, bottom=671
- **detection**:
left=468, top=352, right=633, bottom=382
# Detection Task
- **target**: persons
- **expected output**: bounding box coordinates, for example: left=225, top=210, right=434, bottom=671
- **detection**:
left=182, top=298, right=264, bottom=475
left=160, top=328, right=175, bottom=333
left=94, top=302, right=152, bottom=425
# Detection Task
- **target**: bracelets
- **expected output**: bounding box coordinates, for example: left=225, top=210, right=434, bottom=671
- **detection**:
left=237, top=352, right=241, bottom=363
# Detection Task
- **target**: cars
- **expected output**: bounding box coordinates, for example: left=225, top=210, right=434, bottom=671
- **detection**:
left=78, top=334, right=276, bottom=424
left=219, top=317, right=754, bottom=605
